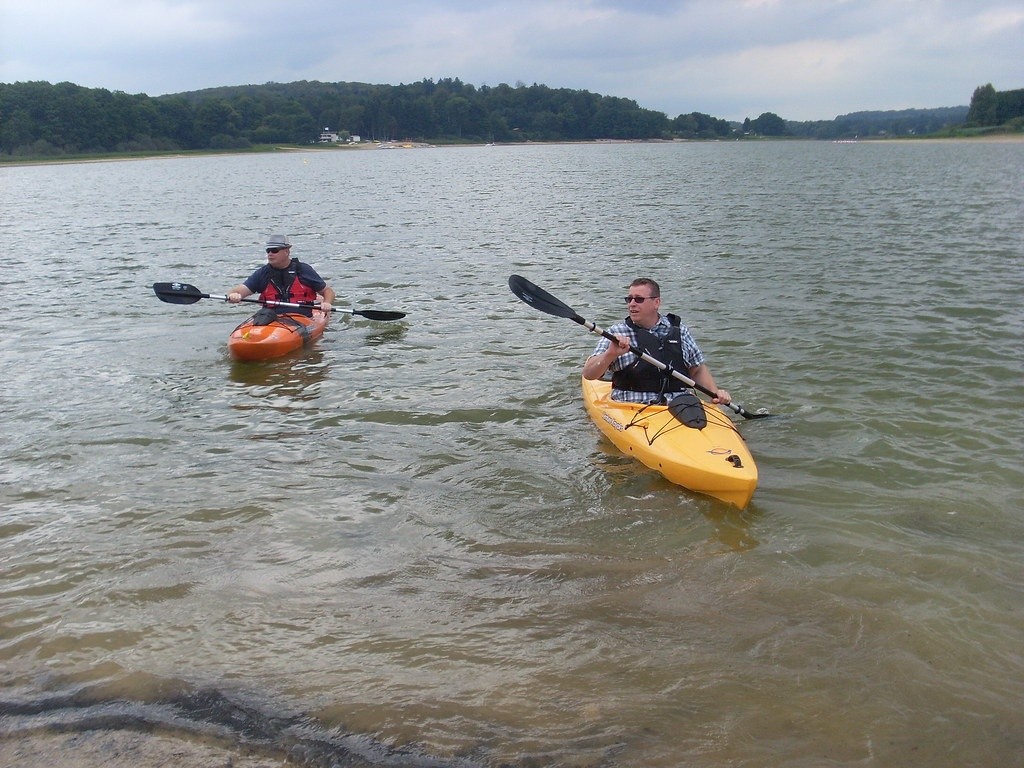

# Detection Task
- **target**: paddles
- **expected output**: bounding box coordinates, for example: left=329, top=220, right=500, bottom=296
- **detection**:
left=507, top=271, right=777, bottom=421
left=151, top=280, right=407, bottom=321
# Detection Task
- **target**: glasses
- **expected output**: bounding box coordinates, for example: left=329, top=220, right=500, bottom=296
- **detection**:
left=624, top=296, right=658, bottom=303
left=266, top=246, right=289, bottom=253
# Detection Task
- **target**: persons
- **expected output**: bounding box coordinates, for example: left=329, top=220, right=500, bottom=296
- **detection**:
left=226, top=235, right=335, bottom=318
left=581, top=278, right=731, bottom=406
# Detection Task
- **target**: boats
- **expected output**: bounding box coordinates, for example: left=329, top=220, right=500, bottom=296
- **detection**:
left=228, top=294, right=329, bottom=362
left=581, top=355, right=758, bottom=511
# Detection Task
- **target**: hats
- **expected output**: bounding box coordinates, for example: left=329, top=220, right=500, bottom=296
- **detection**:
left=265, top=234, right=293, bottom=248
